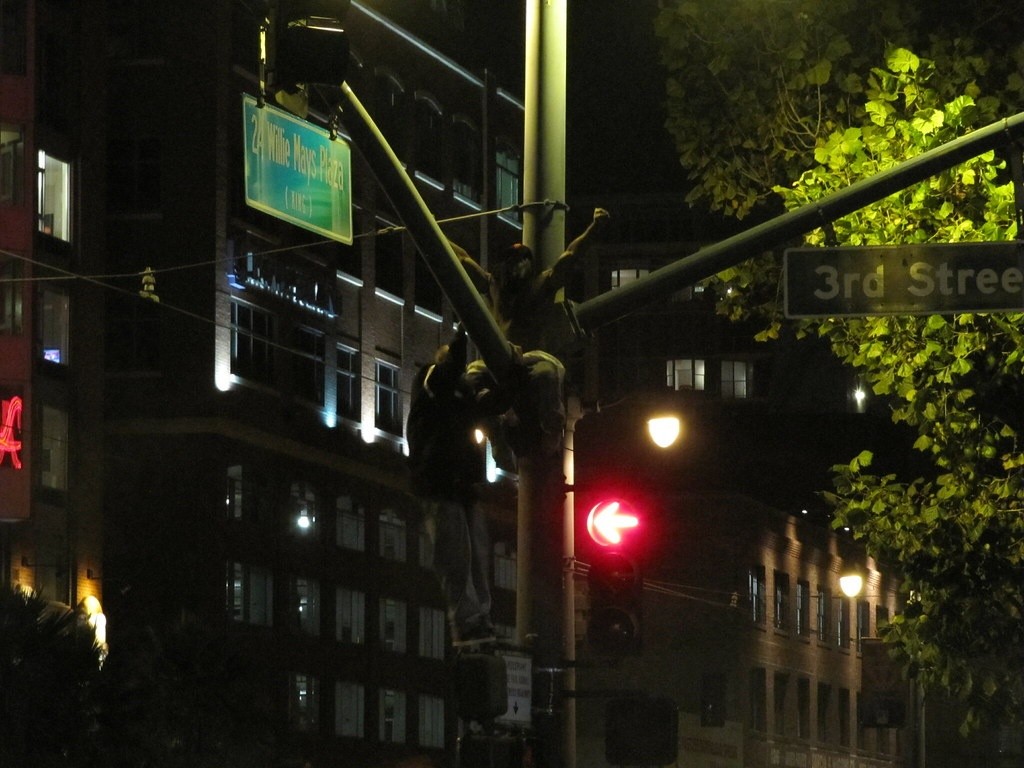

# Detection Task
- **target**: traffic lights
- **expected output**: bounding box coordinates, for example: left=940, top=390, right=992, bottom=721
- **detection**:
left=604, top=695, right=680, bottom=768
left=703, top=673, right=729, bottom=729
left=582, top=469, right=646, bottom=658
left=859, top=636, right=905, bottom=726
